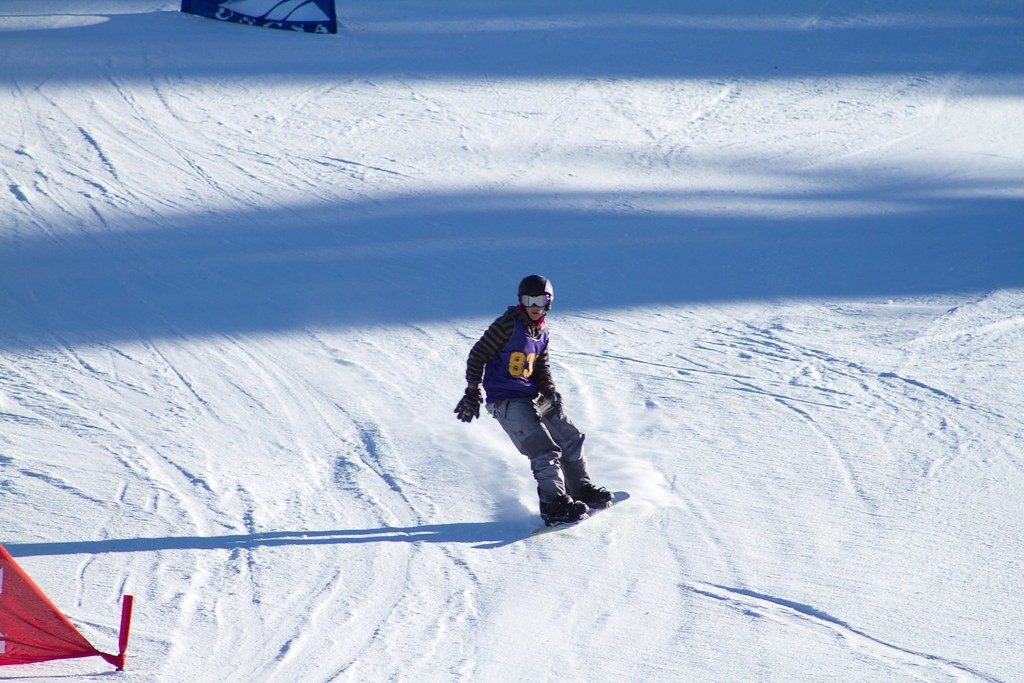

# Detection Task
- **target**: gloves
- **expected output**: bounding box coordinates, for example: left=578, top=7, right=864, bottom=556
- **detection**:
left=454, top=387, right=483, bottom=423
left=544, top=387, right=563, bottom=420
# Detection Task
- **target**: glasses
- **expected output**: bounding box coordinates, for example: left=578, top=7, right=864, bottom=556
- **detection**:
left=519, top=294, right=550, bottom=307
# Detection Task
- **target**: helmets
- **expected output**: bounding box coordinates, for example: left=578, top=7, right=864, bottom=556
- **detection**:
left=517, top=275, right=554, bottom=311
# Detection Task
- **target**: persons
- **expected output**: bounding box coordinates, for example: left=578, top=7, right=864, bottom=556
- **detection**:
left=454, top=274, right=614, bottom=526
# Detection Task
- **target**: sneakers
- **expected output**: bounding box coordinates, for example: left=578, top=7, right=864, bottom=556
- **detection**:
left=566, top=483, right=615, bottom=502
left=540, top=494, right=591, bottom=521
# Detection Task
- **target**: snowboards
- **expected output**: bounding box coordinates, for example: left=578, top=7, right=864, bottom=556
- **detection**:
left=530, top=491, right=630, bottom=536
